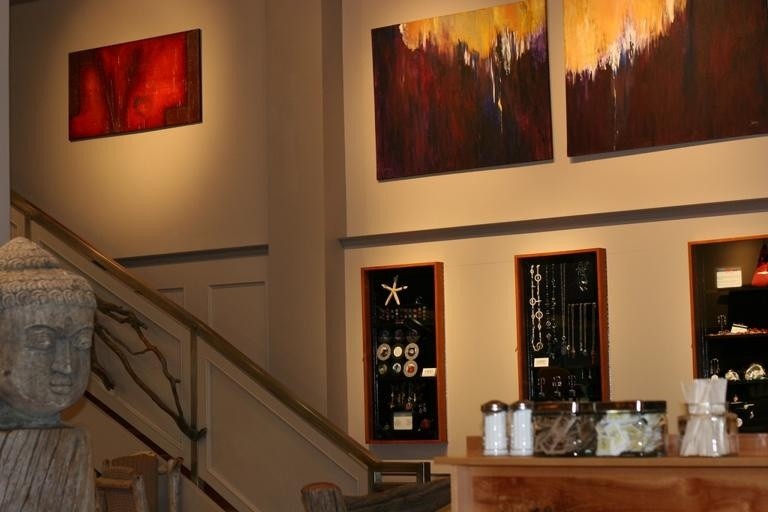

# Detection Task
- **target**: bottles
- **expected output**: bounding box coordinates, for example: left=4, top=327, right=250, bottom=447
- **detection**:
left=511, top=400, right=535, bottom=457
left=482, top=398, right=507, bottom=457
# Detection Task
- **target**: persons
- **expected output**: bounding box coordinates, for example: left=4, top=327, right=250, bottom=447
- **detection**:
left=0, top=236, right=97, bottom=429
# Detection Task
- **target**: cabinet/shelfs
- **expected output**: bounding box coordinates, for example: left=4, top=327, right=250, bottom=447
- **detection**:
left=688, top=234, right=768, bottom=434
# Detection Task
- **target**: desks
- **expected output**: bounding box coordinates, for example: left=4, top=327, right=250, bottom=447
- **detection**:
left=435, top=436, right=766, bottom=510
